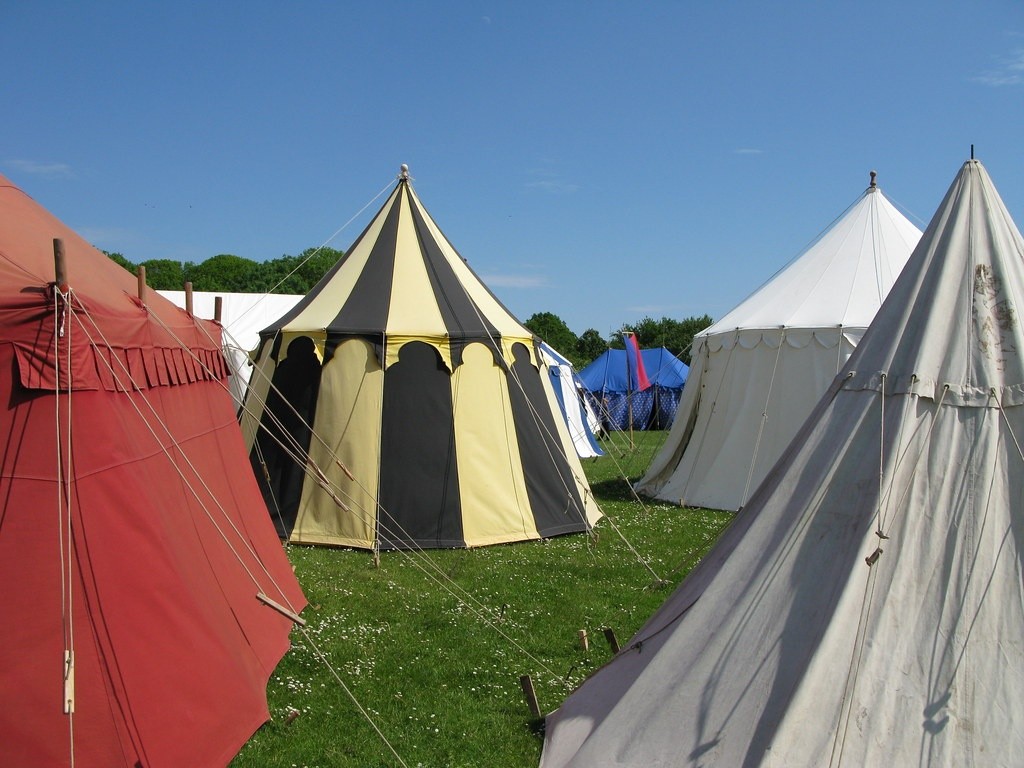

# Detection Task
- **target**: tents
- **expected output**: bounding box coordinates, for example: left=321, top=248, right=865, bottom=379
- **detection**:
left=574, top=380, right=600, bottom=435
left=244, top=162, right=601, bottom=549
left=633, top=169, right=924, bottom=516
left=529, top=340, right=599, bottom=456
left=151, top=289, right=308, bottom=421
left=579, top=339, right=691, bottom=425
left=540, top=144, right=1024, bottom=766
left=1, top=171, right=307, bottom=767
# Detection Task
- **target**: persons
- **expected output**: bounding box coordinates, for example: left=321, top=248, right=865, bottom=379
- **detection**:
left=596, top=398, right=611, bottom=441
